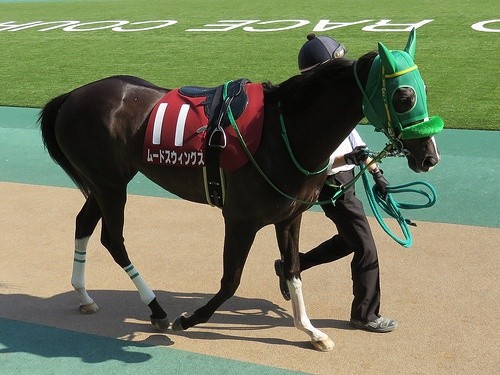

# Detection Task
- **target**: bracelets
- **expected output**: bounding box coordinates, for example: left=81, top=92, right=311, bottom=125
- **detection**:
left=369, top=167, right=378, bottom=175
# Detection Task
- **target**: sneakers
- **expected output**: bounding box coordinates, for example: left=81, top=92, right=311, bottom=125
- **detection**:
left=349, top=314, right=396, bottom=333
left=275, top=259, right=290, bottom=301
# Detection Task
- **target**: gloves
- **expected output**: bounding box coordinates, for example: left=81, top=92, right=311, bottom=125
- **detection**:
left=344, top=146, right=369, bottom=165
left=372, top=168, right=391, bottom=198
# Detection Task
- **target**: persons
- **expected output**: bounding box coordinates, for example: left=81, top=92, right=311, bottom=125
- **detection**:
left=274, top=33, right=399, bottom=334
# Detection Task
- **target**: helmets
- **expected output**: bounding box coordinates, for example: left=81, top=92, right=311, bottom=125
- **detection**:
left=298, top=33, right=347, bottom=72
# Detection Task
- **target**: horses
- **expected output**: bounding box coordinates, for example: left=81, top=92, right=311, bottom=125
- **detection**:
left=34, top=24, right=445, bottom=350
